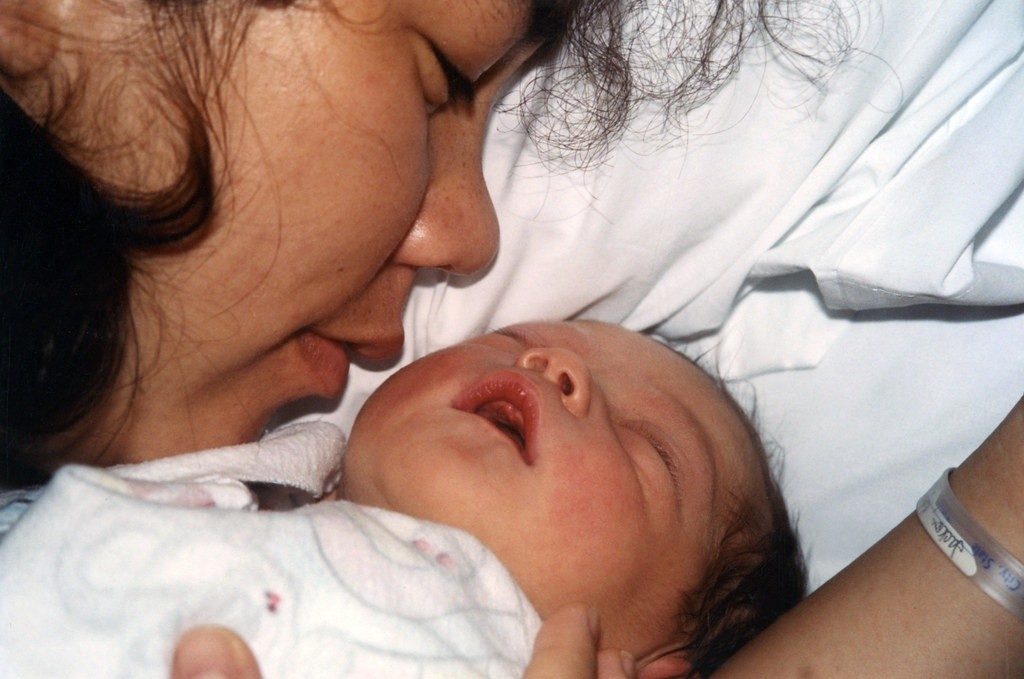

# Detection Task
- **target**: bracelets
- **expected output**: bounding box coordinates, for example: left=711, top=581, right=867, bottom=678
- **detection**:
left=916, top=468, right=1023, bottom=621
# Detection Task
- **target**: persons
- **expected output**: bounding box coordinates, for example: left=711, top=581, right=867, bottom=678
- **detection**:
left=0, top=0, right=1024, bottom=679
left=2, top=314, right=806, bottom=679
